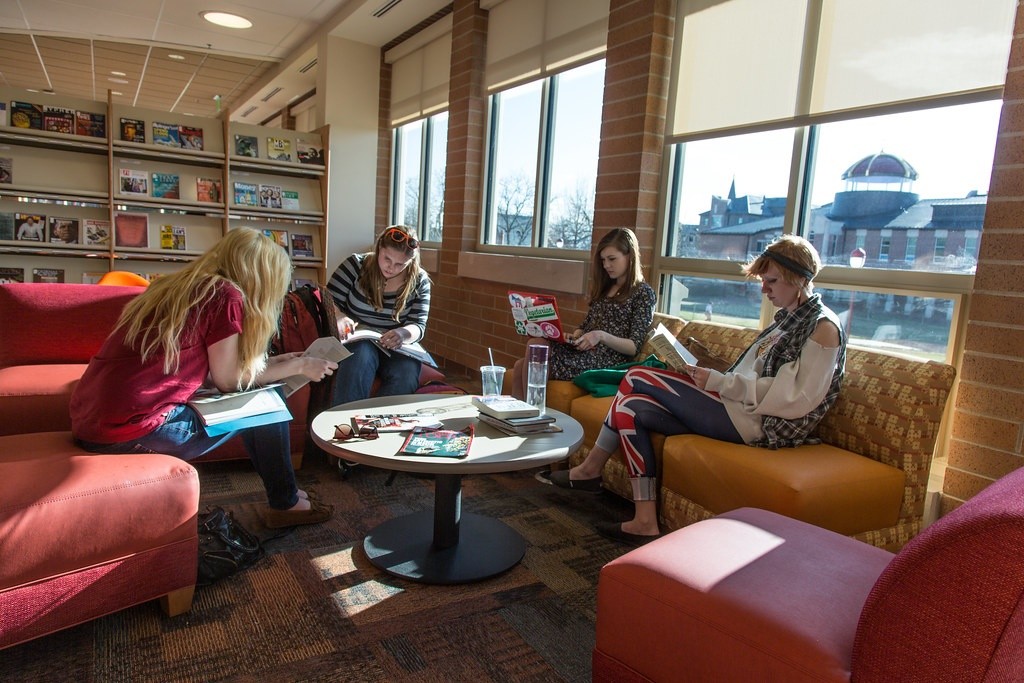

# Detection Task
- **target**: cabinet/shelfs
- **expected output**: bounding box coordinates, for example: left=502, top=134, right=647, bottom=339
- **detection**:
left=0, top=84, right=331, bottom=294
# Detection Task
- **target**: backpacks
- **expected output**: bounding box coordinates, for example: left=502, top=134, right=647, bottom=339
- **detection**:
left=270, top=284, right=330, bottom=356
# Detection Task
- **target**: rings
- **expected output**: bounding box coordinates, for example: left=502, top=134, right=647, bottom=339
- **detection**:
left=693, top=369, right=696, bottom=373
left=692, top=373, right=695, bottom=378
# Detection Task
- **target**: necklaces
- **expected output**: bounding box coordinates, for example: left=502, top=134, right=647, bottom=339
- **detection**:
left=380, top=271, right=403, bottom=289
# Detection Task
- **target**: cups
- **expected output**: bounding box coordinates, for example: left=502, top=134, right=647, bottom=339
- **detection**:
left=526, top=345, right=548, bottom=416
left=480, top=365, right=506, bottom=400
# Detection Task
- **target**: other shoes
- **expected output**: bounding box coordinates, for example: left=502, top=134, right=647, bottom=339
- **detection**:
left=303, top=486, right=317, bottom=501
left=335, top=456, right=353, bottom=481
left=595, top=520, right=661, bottom=547
left=549, top=468, right=604, bottom=495
left=263, top=500, right=336, bottom=528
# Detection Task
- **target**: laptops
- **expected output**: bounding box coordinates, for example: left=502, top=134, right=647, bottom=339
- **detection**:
left=507, top=290, right=597, bottom=350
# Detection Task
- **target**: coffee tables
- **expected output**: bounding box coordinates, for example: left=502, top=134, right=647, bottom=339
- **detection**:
left=310, top=393, right=586, bottom=585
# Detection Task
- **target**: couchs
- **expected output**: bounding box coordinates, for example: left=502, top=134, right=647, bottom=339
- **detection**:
left=1, top=282, right=468, bottom=651
left=592, top=469, right=1024, bottom=683
left=504, top=313, right=957, bottom=554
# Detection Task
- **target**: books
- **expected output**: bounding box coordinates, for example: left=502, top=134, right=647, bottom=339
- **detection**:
left=233, top=134, right=324, bottom=166
left=186, top=383, right=294, bottom=437
left=233, top=182, right=258, bottom=206
left=0, top=101, right=223, bottom=284
left=355, top=395, right=563, bottom=458
left=647, top=322, right=699, bottom=374
left=281, top=191, right=300, bottom=211
left=259, top=184, right=282, bottom=209
left=339, top=324, right=438, bottom=368
left=262, top=229, right=315, bottom=291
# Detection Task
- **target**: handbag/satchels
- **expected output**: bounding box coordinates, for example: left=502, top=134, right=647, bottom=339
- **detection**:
left=198, top=504, right=261, bottom=582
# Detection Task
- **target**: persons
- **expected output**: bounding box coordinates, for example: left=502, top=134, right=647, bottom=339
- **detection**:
left=17, top=217, right=43, bottom=242
left=298, top=147, right=324, bottom=166
left=260, top=189, right=282, bottom=208
left=534, top=233, right=847, bottom=542
left=53, top=220, right=74, bottom=244
left=326, top=225, right=431, bottom=470
left=69, top=226, right=337, bottom=530
left=511, top=228, right=657, bottom=402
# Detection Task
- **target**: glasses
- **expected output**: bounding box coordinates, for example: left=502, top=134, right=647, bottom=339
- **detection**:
left=385, top=228, right=420, bottom=249
